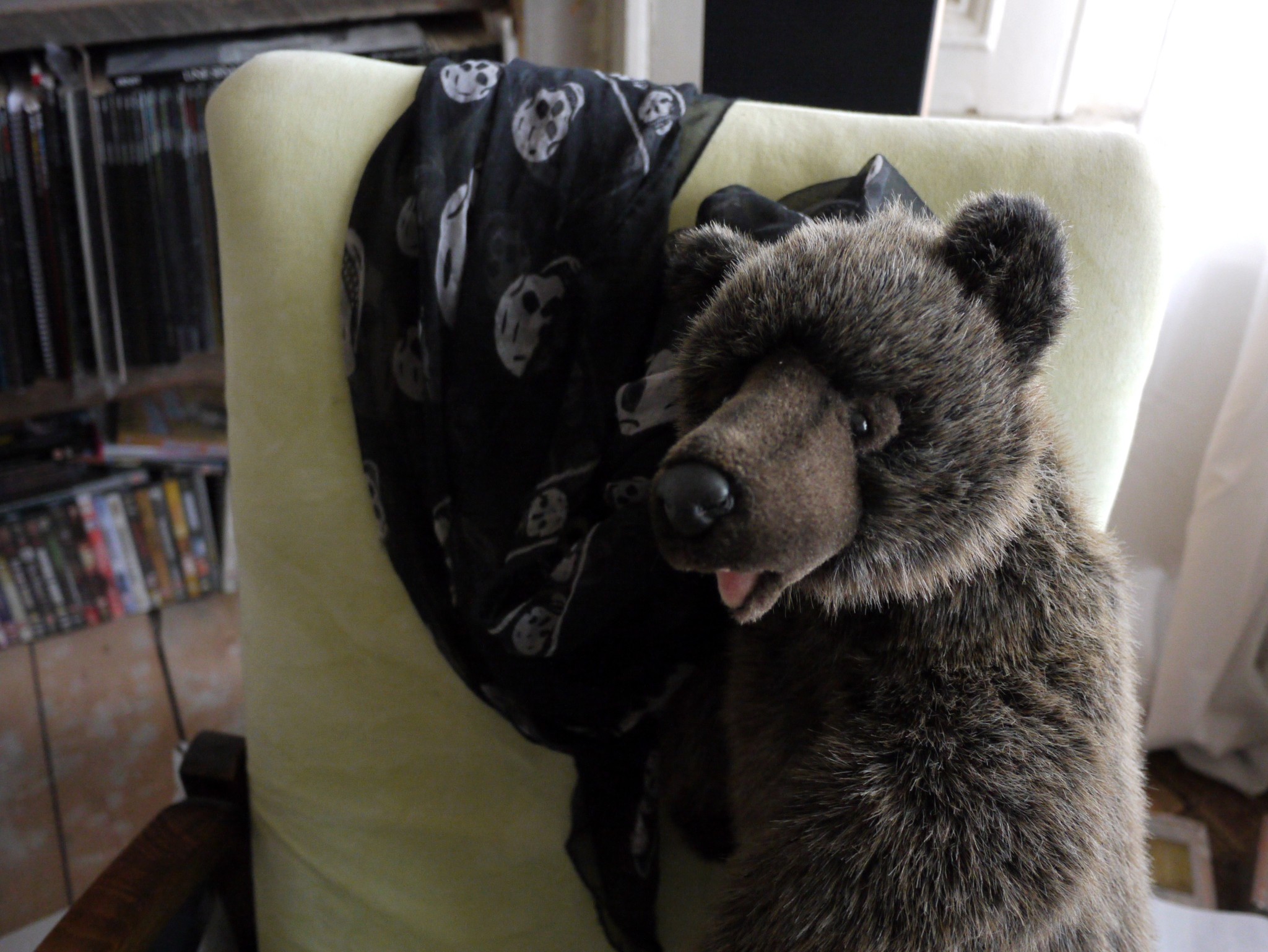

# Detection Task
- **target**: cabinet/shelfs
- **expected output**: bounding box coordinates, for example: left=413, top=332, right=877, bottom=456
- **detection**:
left=1, top=0, right=665, bottom=938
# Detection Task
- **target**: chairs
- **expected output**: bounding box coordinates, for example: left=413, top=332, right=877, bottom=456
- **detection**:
left=24, top=46, right=1268, bottom=951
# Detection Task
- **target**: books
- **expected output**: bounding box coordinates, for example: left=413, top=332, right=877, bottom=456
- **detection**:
left=0, top=385, right=238, bottom=643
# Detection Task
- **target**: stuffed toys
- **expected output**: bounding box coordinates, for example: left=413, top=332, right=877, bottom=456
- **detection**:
left=657, top=190, right=1162, bottom=952
